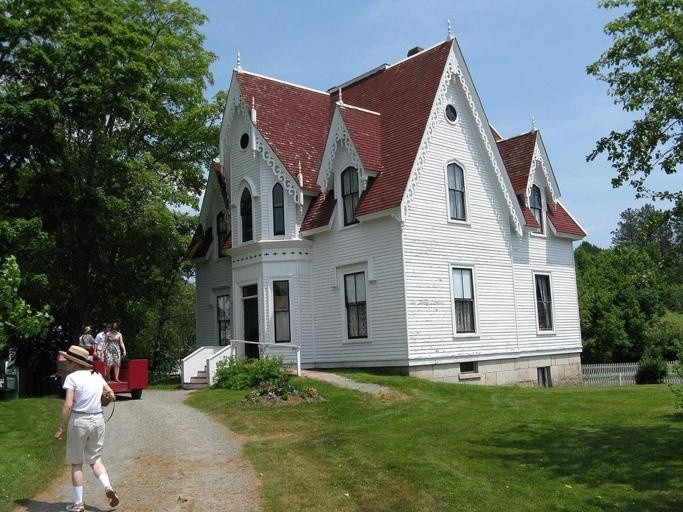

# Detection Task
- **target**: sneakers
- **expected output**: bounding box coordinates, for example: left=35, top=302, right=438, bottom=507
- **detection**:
left=105, top=488, right=120, bottom=507
left=65, top=501, right=85, bottom=512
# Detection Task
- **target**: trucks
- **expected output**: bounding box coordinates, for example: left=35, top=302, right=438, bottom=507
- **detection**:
left=54, top=352, right=148, bottom=401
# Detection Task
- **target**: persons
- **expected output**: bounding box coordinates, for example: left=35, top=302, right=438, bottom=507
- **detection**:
left=77, top=325, right=98, bottom=349
left=99, top=321, right=127, bottom=383
left=92, top=322, right=111, bottom=380
left=50, top=345, right=120, bottom=512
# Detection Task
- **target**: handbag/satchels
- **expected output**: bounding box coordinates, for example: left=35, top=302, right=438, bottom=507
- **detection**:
left=101, top=391, right=113, bottom=407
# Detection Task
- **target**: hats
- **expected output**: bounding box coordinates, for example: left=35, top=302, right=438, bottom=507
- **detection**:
left=57, top=344, right=95, bottom=368
left=83, top=326, right=92, bottom=334
